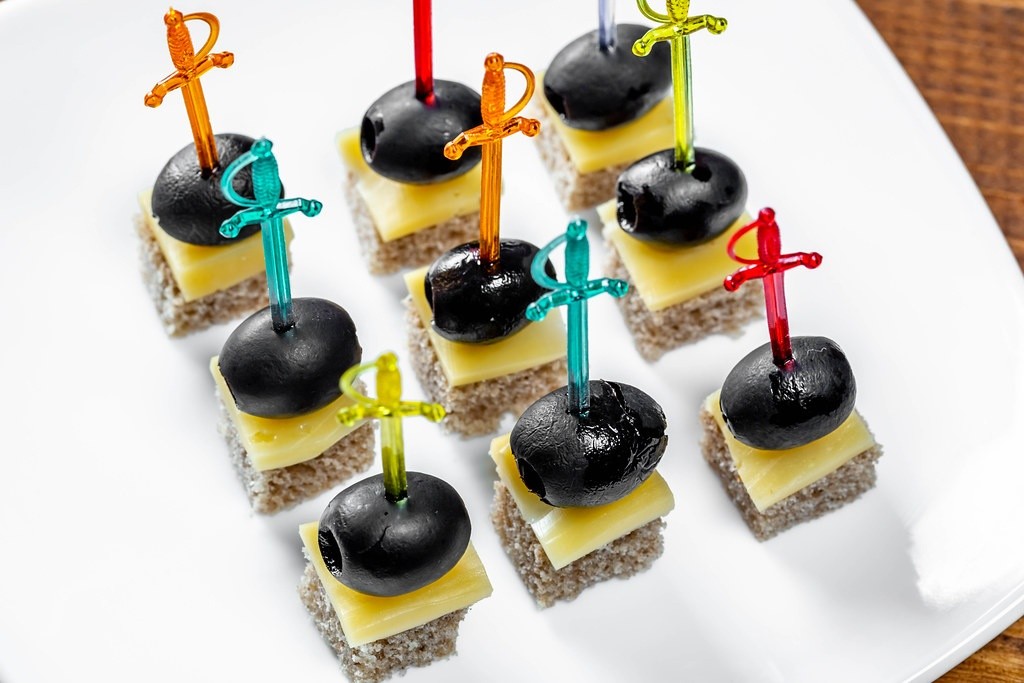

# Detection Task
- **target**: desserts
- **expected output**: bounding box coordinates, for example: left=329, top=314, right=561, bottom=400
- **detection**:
left=136, top=0, right=884, bottom=683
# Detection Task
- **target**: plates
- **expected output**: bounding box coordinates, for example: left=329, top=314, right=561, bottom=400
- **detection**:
left=0, top=0, right=1024, bottom=683
